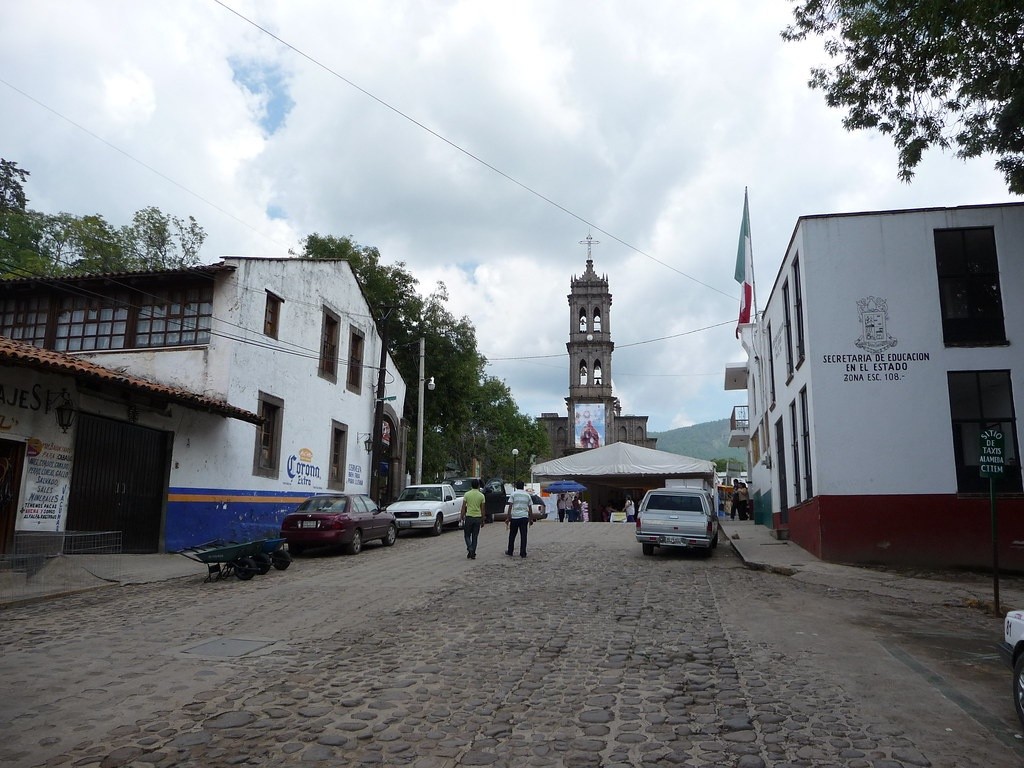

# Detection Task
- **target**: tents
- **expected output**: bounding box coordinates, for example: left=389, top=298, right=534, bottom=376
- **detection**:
left=531, top=442, right=718, bottom=518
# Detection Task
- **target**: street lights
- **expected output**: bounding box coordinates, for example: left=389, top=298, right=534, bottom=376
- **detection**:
left=512, top=449, right=519, bottom=491
left=415, top=376, right=437, bottom=487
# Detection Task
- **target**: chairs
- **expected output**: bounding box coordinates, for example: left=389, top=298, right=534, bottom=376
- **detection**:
left=323, top=499, right=333, bottom=508
left=416, top=493, right=425, bottom=498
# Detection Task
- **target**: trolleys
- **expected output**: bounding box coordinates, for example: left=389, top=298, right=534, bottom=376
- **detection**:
left=174, top=537, right=293, bottom=584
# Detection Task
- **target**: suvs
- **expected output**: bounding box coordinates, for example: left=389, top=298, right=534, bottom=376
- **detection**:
left=441, top=476, right=507, bottom=518
left=635, top=488, right=719, bottom=558
left=494, top=494, right=548, bottom=526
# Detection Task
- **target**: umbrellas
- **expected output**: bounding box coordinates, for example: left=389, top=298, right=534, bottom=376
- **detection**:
left=542, top=480, right=587, bottom=515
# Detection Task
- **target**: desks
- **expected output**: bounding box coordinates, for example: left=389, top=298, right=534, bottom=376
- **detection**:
left=610, top=512, right=627, bottom=523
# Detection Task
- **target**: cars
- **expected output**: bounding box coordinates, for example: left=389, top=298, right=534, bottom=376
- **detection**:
left=280, top=494, right=397, bottom=556
left=995, top=610, right=1024, bottom=730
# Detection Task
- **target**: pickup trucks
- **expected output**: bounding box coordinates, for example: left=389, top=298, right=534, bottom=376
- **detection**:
left=386, top=484, right=467, bottom=537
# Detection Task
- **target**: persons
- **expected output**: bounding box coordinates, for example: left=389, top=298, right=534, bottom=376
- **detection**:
left=460, top=479, right=486, bottom=559
left=557, top=493, right=584, bottom=522
left=604, top=496, right=645, bottom=523
left=730, top=478, right=753, bottom=521
left=503, top=480, right=533, bottom=558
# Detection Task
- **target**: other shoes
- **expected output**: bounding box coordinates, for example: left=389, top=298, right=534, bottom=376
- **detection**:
left=467, top=553, right=476, bottom=558
left=521, top=555, right=527, bottom=560
left=470, top=552, right=475, bottom=559
left=505, top=550, right=513, bottom=556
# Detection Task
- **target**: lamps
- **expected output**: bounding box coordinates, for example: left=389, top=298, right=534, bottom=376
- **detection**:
left=356, top=432, right=374, bottom=453
left=45, top=387, right=79, bottom=434
